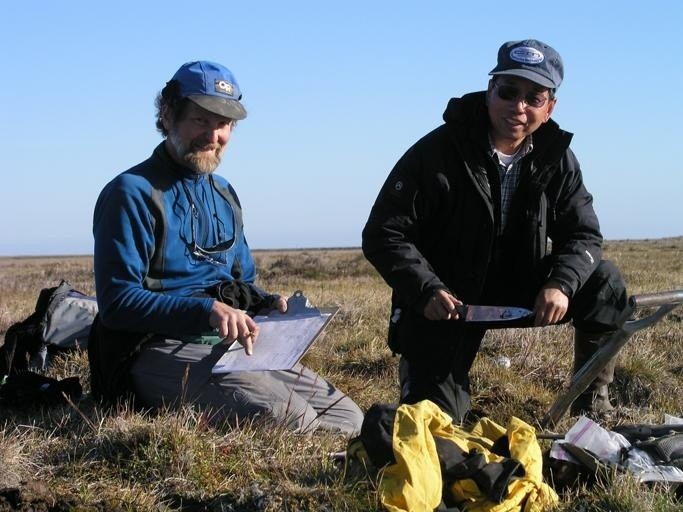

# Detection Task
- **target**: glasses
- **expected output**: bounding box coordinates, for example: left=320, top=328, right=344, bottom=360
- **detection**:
left=492, top=82, right=554, bottom=108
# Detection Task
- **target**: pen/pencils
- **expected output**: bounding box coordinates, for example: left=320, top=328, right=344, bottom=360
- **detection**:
left=249, top=331, right=255, bottom=337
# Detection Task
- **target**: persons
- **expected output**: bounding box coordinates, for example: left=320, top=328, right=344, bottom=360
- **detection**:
left=360, top=38, right=631, bottom=434
left=85, top=60, right=366, bottom=440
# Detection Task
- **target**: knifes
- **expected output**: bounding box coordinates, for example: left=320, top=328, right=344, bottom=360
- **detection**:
left=456, top=305, right=533, bottom=322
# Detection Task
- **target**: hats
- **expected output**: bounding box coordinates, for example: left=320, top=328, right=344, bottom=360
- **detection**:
left=486, top=38, right=566, bottom=98
left=166, top=59, right=248, bottom=128
